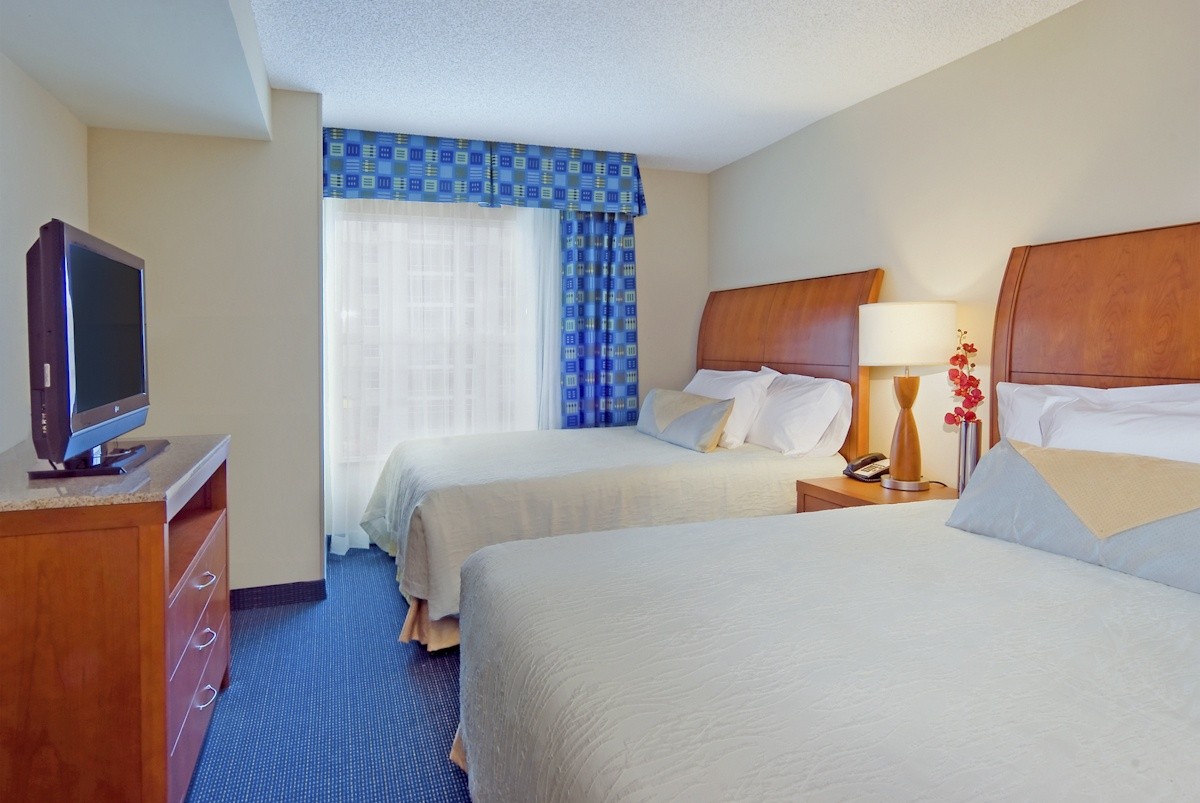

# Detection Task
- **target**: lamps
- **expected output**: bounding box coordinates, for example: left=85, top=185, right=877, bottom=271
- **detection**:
left=857, top=300, right=961, bottom=493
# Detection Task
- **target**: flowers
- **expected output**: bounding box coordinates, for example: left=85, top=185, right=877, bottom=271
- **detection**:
left=946, top=328, right=985, bottom=423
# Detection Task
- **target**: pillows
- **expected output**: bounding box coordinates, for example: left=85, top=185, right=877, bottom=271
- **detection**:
left=994, top=380, right=1200, bottom=462
left=745, top=365, right=852, bottom=457
left=945, top=440, right=1199, bottom=595
left=637, top=388, right=735, bottom=453
left=680, top=368, right=777, bottom=449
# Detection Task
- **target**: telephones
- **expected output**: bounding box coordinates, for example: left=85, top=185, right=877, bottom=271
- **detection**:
left=843, top=452, right=890, bottom=480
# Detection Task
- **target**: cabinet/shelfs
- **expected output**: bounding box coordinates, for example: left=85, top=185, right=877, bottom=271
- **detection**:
left=0, top=435, right=232, bottom=803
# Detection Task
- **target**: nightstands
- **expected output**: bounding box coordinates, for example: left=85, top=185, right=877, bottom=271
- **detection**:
left=797, top=477, right=960, bottom=513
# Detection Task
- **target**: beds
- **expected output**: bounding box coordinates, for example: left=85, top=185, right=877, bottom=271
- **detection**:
left=360, top=268, right=885, bottom=652
left=450, top=219, right=1200, bottom=803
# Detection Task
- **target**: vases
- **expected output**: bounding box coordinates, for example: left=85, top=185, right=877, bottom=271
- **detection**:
left=957, top=421, right=980, bottom=498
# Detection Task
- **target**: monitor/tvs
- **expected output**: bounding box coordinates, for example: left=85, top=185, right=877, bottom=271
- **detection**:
left=25, top=218, right=149, bottom=472
